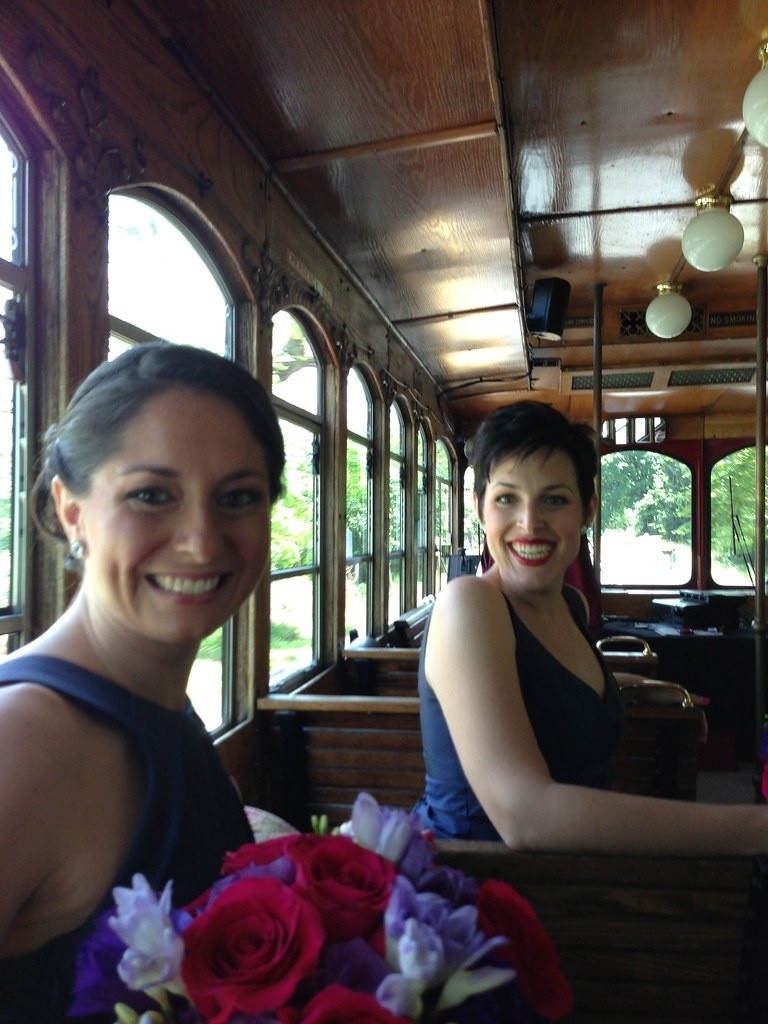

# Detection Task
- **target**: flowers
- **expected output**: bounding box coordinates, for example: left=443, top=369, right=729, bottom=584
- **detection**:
left=97, top=790, right=574, bottom=1024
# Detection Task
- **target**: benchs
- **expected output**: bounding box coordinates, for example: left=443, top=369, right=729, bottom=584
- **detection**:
left=255, top=690, right=709, bottom=831
left=340, top=646, right=658, bottom=700
left=423, top=837, right=767, bottom=1024
left=394, top=602, right=433, bottom=649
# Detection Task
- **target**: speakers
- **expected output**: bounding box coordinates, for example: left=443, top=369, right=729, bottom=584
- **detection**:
left=527, top=277, right=571, bottom=341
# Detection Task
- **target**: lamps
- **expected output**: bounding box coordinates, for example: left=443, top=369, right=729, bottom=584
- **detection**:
left=643, top=282, right=695, bottom=339
left=681, top=192, right=744, bottom=274
left=740, top=35, right=767, bottom=148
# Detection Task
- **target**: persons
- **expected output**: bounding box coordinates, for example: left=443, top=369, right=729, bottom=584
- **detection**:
left=412, top=397, right=767, bottom=859
left=1, top=341, right=285, bottom=1024
